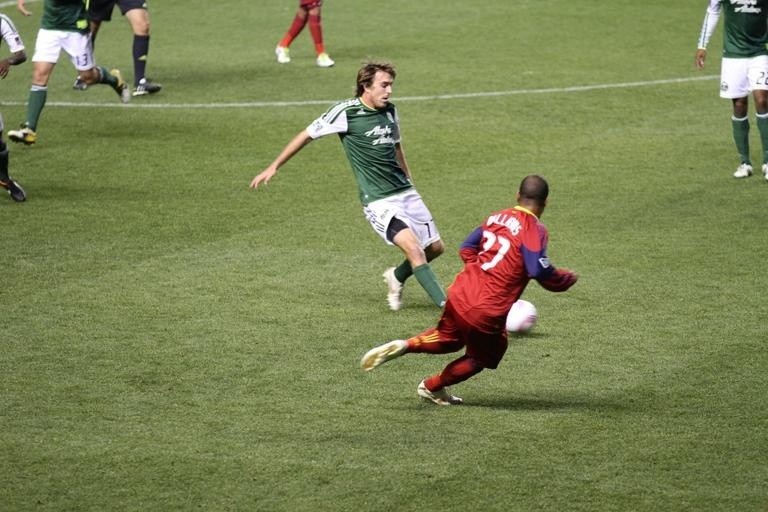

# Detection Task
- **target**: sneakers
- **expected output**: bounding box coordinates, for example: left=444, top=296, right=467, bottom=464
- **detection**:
left=316, top=52, right=334, bottom=68
left=7, top=121, right=36, bottom=145
left=734, top=163, right=753, bottom=178
left=110, top=68, right=131, bottom=103
left=132, top=75, right=161, bottom=97
left=361, top=340, right=406, bottom=372
left=275, top=43, right=291, bottom=63
left=417, top=380, right=463, bottom=405
left=0, top=178, right=26, bottom=203
left=382, top=266, right=405, bottom=311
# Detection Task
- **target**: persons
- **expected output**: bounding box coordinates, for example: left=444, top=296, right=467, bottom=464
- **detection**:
left=72, top=0, right=163, bottom=97
left=692, top=0, right=768, bottom=181
left=7, top=0, right=133, bottom=146
left=247, top=59, right=450, bottom=314
left=358, top=174, right=581, bottom=406
left=0, top=13, right=29, bottom=203
left=274, top=0, right=335, bottom=70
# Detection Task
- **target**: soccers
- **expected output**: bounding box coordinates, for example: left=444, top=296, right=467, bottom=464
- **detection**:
left=505, top=299, right=537, bottom=333
